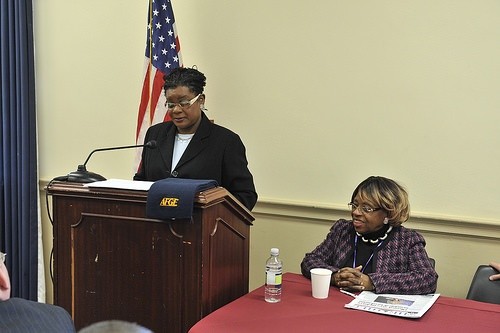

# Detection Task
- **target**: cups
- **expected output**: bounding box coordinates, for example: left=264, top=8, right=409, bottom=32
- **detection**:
left=310, top=268, right=332, bottom=299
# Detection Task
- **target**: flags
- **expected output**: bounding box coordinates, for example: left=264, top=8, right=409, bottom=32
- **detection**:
left=136, top=0, right=183, bottom=172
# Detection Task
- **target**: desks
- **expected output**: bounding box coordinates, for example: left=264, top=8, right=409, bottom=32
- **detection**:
left=188, top=273, right=500, bottom=333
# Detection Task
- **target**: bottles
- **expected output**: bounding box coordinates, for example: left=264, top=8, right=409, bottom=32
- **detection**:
left=264, top=248, right=282, bottom=302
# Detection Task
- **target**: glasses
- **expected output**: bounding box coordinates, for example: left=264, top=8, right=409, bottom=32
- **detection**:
left=164, top=94, right=200, bottom=109
left=0, top=253, right=7, bottom=262
left=348, top=201, right=381, bottom=214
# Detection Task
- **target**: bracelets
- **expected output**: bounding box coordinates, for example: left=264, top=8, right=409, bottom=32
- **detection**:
left=355, top=226, right=394, bottom=243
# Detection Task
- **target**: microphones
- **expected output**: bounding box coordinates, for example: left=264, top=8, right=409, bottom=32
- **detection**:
left=67, top=140, right=159, bottom=182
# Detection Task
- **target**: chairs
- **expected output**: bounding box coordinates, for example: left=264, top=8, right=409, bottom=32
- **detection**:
left=466, top=266, right=500, bottom=303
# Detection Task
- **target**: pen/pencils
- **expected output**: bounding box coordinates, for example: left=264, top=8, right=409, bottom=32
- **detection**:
left=339, top=289, right=357, bottom=298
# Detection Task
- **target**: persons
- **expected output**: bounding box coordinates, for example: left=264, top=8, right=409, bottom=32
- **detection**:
left=133, top=65, right=258, bottom=212
left=489, top=262, right=500, bottom=281
left=301, top=176, right=439, bottom=294
left=0, top=252, right=76, bottom=333
left=78, top=321, right=154, bottom=333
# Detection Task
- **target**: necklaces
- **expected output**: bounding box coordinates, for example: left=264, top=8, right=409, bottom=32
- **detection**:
left=353, top=234, right=383, bottom=273
left=177, top=133, right=190, bottom=142
